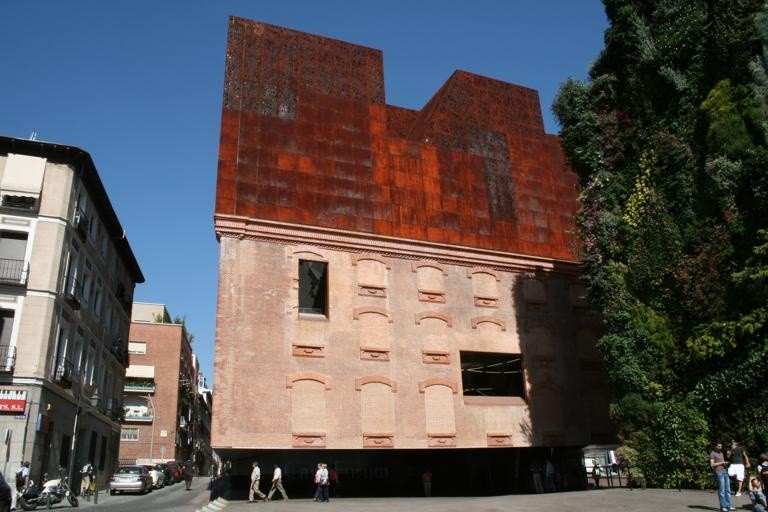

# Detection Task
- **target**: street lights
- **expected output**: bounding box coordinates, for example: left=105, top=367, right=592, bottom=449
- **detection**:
left=68, top=393, right=101, bottom=489
left=138, top=394, right=156, bottom=463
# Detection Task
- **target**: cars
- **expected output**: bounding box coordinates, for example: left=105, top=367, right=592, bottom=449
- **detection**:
left=109, top=461, right=182, bottom=496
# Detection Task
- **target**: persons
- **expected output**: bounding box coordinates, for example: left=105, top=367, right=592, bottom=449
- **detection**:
left=80, top=464, right=98, bottom=493
left=248, top=461, right=266, bottom=503
left=749, top=453, right=768, bottom=512
left=313, top=463, right=338, bottom=502
left=15, top=459, right=32, bottom=512
left=422, top=468, right=433, bottom=497
left=265, top=462, right=289, bottom=502
left=182, top=458, right=195, bottom=490
left=592, top=463, right=603, bottom=487
left=709, top=440, right=737, bottom=512
left=727, top=438, right=751, bottom=496
left=531, top=458, right=556, bottom=493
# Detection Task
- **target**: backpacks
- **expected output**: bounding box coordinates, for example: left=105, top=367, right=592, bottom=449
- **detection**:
left=760, top=464, right=768, bottom=482
left=16, top=467, right=28, bottom=487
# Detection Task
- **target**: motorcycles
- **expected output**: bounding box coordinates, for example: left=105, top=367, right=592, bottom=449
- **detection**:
left=16, top=464, right=78, bottom=510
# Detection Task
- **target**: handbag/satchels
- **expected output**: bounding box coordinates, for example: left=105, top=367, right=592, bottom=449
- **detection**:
left=320, top=480, right=330, bottom=486
